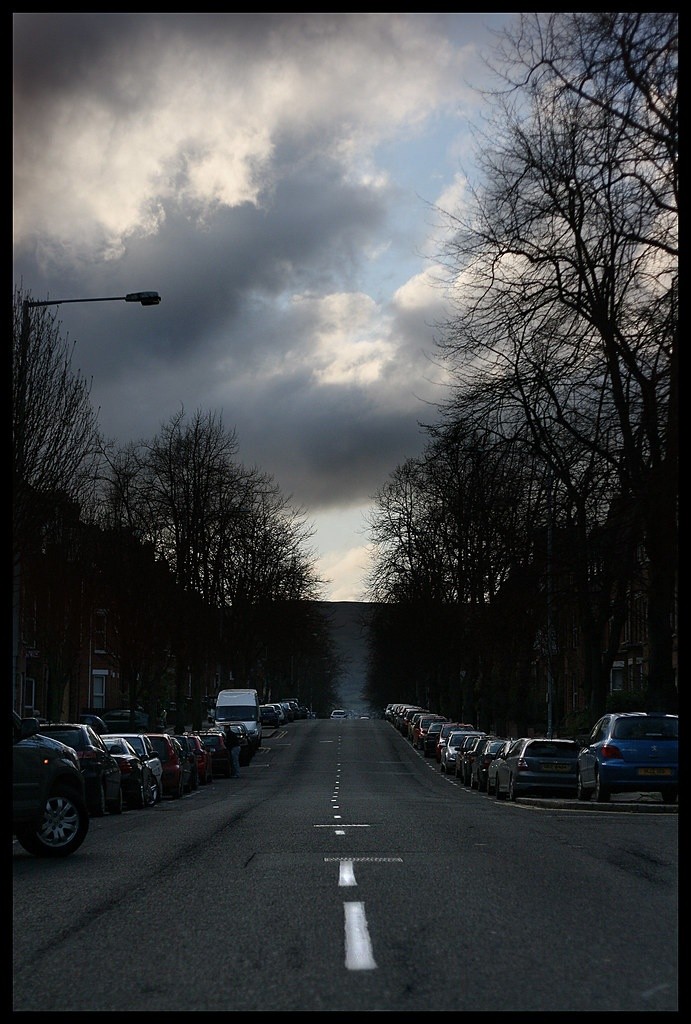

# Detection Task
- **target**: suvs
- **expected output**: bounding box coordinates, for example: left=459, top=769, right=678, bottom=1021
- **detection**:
left=10, top=710, right=90, bottom=861
left=30, top=723, right=125, bottom=815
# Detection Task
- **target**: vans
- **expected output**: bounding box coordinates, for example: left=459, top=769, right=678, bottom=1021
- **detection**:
left=207, top=689, right=263, bottom=743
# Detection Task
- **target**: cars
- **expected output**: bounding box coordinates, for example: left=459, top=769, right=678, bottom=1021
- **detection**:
left=259, top=705, right=280, bottom=729
left=267, top=698, right=317, bottom=724
left=144, top=734, right=186, bottom=799
left=495, top=737, right=582, bottom=802
left=361, top=715, right=370, bottom=720
left=99, top=733, right=164, bottom=791
left=383, top=703, right=515, bottom=793
left=102, top=737, right=163, bottom=810
left=331, top=710, right=347, bottom=720
left=180, top=725, right=254, bottom=793
left=574, top=713, right=679, bottom=802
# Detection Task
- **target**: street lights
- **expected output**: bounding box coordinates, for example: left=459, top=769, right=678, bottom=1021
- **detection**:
left=9, top=290, right=163, bottom=672
